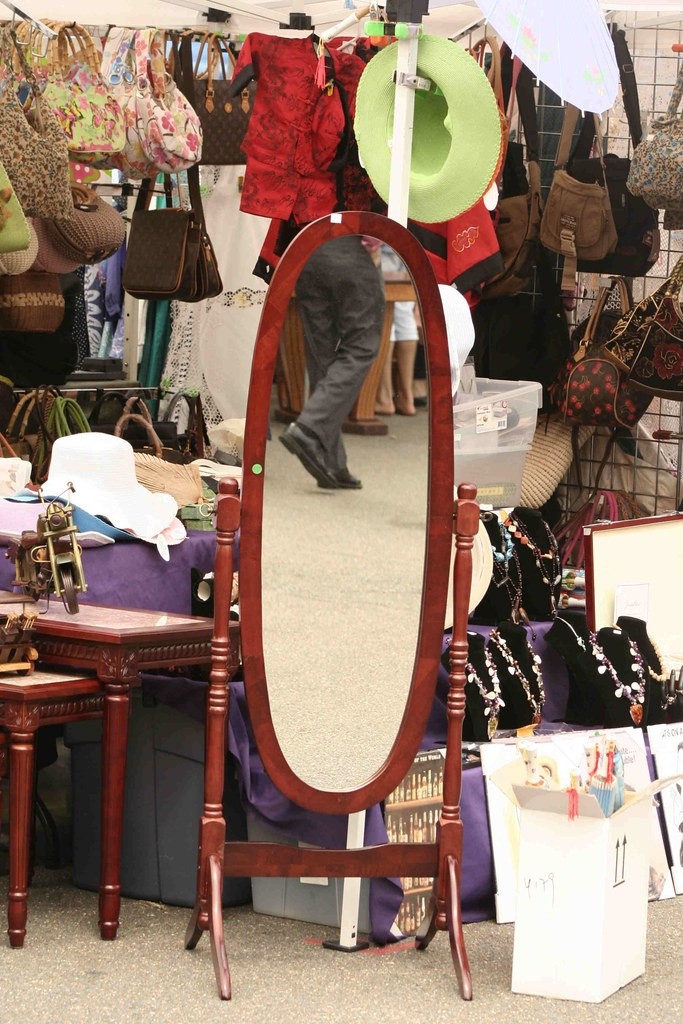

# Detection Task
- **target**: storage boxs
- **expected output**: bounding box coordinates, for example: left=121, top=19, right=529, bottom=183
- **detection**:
left=61, top=686, right=680, bottom=1005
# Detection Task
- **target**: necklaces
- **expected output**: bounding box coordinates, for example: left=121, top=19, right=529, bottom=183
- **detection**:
left=588, top=630, right=646, bottom=726
left=556, top=616, right=588, bottom=652
left=614, top=622, right=667, bottom=683
left=444, top=637, right=506, bottom=739
left=488, top=629, right=545, bottom=724
left=490, top=521, right=531, bottom=627
left=505, top=510, right=562, bottom=620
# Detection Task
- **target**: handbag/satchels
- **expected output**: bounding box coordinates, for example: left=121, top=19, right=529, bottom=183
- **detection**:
left=473, top=30, right=683, bottom=610
left=0, top=20, right=257, bottom=385
left=123, top=206, right=223, bottom=303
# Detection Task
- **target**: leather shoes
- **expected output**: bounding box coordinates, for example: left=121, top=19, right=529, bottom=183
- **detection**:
left=317, top=471, right=361, bottom=489
left=280, top=423, right=339, bottom=490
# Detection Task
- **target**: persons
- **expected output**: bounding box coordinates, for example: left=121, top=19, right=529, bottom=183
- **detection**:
left=279, top=233, right=387, bottom=490
left=374, top=243, right=419, bottom=416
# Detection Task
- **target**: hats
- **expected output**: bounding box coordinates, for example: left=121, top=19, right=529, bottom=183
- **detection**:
left=0, top=392, right=209, bottom=547
left=354, top=35, right=508, bottom=225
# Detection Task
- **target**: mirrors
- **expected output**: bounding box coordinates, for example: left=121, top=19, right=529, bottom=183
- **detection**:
left=186, top=210, right=479, bottom=998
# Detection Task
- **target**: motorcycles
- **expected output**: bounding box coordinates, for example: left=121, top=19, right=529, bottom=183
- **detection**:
left=4, top=482, right=89, bottom=614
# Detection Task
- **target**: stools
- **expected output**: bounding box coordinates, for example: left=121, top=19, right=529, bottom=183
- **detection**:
left=0, top=669, right=105, bottom=948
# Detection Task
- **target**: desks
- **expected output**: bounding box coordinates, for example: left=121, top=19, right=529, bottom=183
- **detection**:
left=0, top=523, right=243, bottom=618
left=0, top=589, right=242, bottom=941
